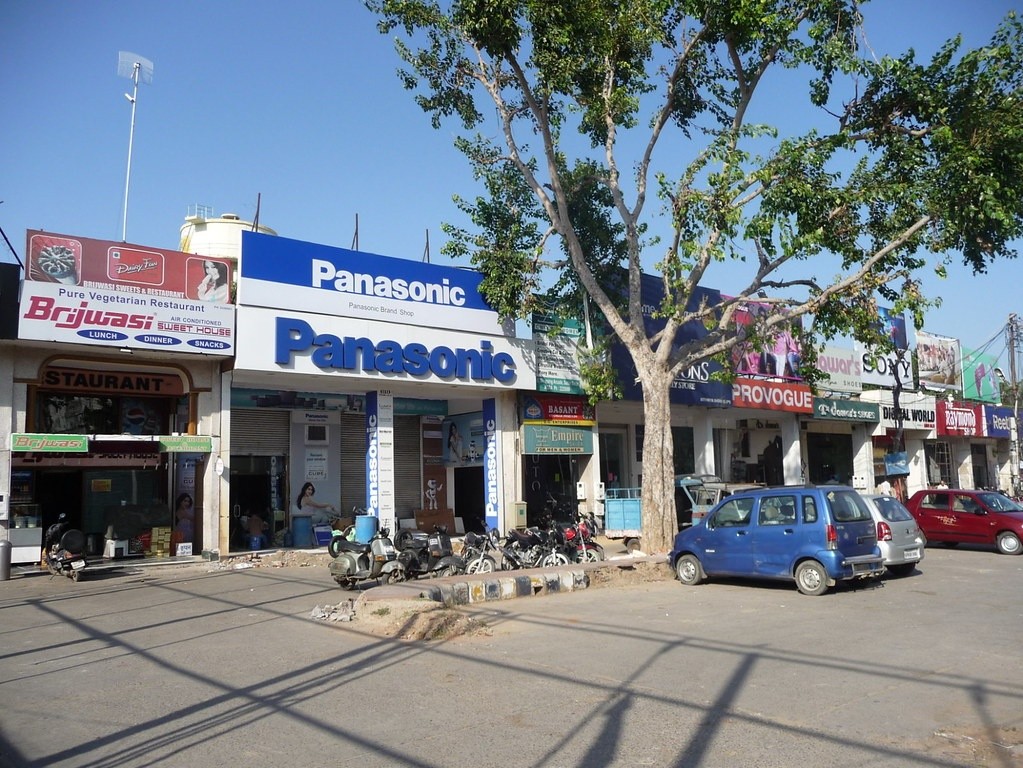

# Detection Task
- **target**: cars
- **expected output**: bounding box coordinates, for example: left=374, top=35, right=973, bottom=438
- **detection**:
left=784, top=495, right=927, bottom=575
left=904, top=490, right=1023, bottom=555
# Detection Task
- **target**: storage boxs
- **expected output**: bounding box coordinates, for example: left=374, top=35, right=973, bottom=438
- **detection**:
left=175, top=542, right=193, bottom=557
left=335, top=516, right=352, bottom=536
left=144, top=525, right=170, bottom=557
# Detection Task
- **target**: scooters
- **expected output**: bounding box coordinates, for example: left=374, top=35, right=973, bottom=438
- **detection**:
left=394, top=524, right=463, bottom=581
left=44, top=513, right=89, bottom=582
left=327, top=527, right=405, bottom=590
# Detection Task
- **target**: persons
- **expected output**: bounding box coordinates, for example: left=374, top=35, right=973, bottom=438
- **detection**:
left=447, top=422, right=464, bottom=466
left=297, top=482, right=340, bottom=523
left=937, top=480, right=949, bottom=489
left=198, top=260, right=228, bottom=302
left=175, top=493, right=195, bottom=526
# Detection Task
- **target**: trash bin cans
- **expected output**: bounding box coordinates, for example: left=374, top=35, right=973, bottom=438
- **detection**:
left=292, top=515, right=315, bottom=549
left=355, top=515, right=376, bottom=545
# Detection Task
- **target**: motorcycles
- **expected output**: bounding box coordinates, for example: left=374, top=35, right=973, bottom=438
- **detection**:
left=459, top=512, right=604, bottom=575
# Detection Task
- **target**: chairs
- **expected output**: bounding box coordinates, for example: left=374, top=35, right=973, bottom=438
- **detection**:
left=765, top=506, right=780, bottom=526
left=779, top=505, right=795, bottom=525
left=954, top=498, right=965, bottom=512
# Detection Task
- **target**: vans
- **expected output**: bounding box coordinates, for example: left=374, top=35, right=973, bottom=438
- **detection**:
left=669, top=483, right=885, bottom=596
left=681, top=475, right=785, bottom=527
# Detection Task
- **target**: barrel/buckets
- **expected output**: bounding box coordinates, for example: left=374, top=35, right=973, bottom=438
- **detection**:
left=292, top=514, right=314, bottom=549
left=354, top=515, right=378, bottom=545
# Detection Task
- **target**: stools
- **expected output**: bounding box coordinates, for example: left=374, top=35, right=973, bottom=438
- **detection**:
left=109, top=540, right=130, bottom=558
